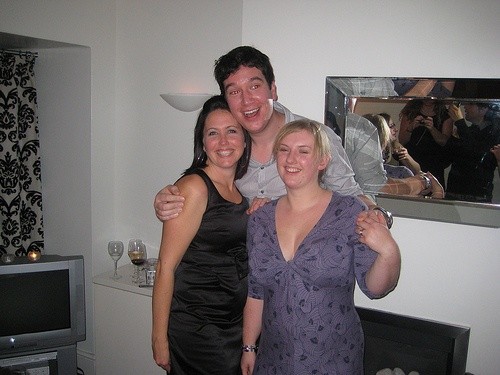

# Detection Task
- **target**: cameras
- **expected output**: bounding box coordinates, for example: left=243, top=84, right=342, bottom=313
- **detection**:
left=419, top=117, right=428, bottom=125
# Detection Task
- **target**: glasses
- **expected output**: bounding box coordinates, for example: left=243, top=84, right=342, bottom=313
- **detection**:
left=388, top=123, right=395, bottom=128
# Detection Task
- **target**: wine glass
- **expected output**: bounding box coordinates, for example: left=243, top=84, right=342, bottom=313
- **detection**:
left=128, top=239, right=142, bottom=281
left=108, top=241, right=124, bottom=279
left=130, top=242, right=147, bottom=283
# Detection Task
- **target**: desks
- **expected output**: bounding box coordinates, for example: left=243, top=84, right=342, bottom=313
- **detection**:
left=91, top=260, right=167, bottom=375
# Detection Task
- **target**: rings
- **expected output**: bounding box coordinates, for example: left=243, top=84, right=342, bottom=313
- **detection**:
left=360, top=230, right=364, bottom=236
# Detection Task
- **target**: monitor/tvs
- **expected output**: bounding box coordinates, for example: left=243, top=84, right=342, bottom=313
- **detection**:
left=0, top=251, right=86, bottom=361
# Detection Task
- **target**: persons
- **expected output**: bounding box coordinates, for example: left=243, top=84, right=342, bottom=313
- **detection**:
left=153, top=46, right=388, bottom=229
left=240, top=119, right=400, bottom=375
left=151, top=96, right=271, bottom=375
left=327, top=77, right=500, bottom=206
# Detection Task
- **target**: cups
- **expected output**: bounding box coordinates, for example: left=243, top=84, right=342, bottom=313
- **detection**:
left=145, top=258, right=159, bottom=285
left=391, top=146, right=402, bottom=162
left=452, top=100, right=461, bottom=107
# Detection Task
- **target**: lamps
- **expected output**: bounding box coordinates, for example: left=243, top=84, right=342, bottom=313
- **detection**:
left=158, top=94, right=216, bottom=112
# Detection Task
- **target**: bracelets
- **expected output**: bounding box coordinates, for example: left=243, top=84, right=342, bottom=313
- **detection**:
left=241, top=344, right=258, bottom=353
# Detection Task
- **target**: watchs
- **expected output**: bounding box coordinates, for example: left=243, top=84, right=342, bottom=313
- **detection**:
left=373, top=206, right=393, bottom=230
left=420, top=175, right=430, bottom=189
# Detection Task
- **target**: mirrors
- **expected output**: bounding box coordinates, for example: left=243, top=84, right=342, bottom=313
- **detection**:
left=324, top=76, right=500, bottom=228
